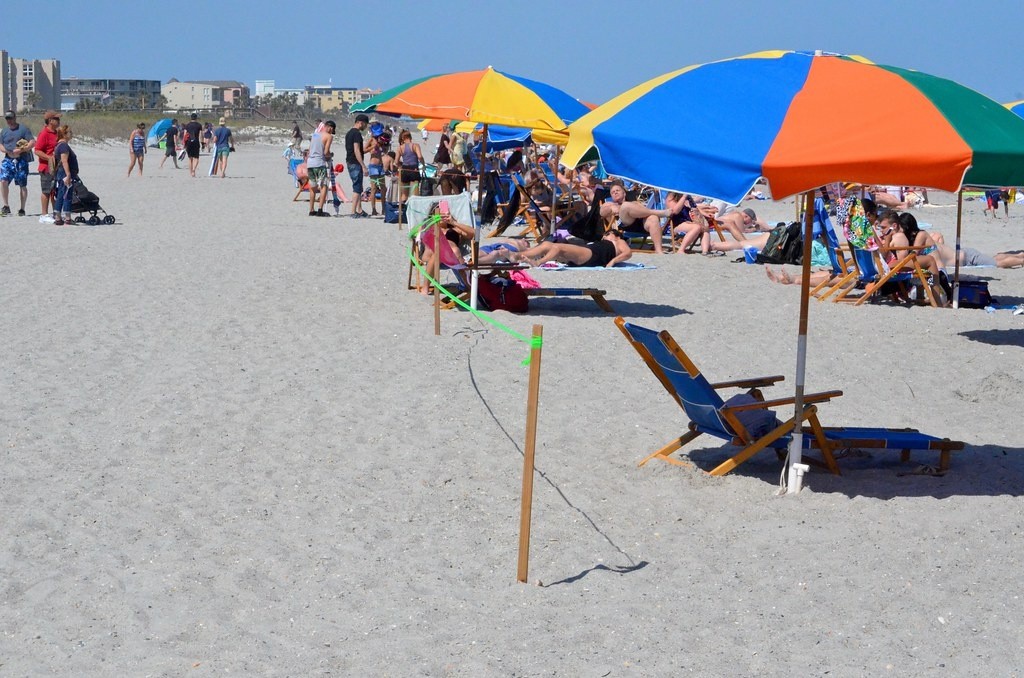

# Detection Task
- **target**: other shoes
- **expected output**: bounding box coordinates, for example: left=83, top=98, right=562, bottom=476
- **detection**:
left=309, top=210, right=329, bottom=217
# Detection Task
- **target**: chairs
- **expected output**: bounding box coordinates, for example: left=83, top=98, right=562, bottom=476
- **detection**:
left=808, top=197, right=859, bottom=301
left=402, top=158, right=726, bottom=312
left=608, top=313, right=965, bottom=478
left=286, top=156, right=341, bottom=204
left=833, top=197, right=948, bottom=310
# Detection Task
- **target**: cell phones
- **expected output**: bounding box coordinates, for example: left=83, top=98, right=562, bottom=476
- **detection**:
left=62, top=177, right=73, bottom=189
left=885, top=227, right=894, bottom=235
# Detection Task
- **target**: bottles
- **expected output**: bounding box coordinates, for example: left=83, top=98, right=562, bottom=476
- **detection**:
left=983, top=306, right=995, bottom=314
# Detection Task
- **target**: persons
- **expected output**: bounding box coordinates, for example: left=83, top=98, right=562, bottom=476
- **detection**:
left=127, top=121, right=147, bottom=177
left=155, top=112, right=235, bottom=179
left=0, top=110, right=80, bottom=225
left=286, top=116, right=1024, bottom=307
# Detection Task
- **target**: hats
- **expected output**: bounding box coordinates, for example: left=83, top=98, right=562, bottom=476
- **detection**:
left=219, top=116, right=226, bottom=124
left=326, top=120, right=336, bottom=135
left=356, top=114, right=371, bottom=124
left=744, top=208, right=757, bottom=222
left=5, top=111, right=15, bottom=118
left=190, top=113, right=197, bottom=120
left=371, top=122, right=384, bottom=136
left=44, top=109, right=63, bottom=119
left=369, top=117, right=379, bottom=123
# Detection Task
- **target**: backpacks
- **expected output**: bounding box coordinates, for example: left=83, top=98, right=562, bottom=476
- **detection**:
left=760, top=221, right=803, bottom=264
left=48, top=142, right=70, bottom=177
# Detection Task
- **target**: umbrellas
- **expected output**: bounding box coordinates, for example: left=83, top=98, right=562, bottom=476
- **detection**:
left=146, top=117, right=176, bottom=146
left=560, top=48, right=1024, bottom=493
left=347, top=65, right=602, bottom=243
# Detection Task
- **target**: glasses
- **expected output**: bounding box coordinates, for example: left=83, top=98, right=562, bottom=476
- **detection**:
left=138, top=126, right=145, bottom=129
left=865, top=210, right=879, bottom=219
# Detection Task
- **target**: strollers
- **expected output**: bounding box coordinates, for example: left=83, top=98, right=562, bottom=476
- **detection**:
left=67, top=175, right=116, bottom=226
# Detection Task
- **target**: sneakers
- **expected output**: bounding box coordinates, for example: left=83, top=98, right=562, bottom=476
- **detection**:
left=350, top=210, right=371, bottom=219
left=0, top=205, right=76, bottom=225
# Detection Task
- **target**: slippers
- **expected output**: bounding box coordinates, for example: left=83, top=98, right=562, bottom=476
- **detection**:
left=702, top=249, right=727, bottom=257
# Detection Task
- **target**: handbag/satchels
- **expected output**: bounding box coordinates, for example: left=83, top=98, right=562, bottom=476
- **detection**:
left=384, top=200, right=408, bottom=224
left=478, top=272, right=529, bottom=314
left=420, top=171, right=435, bottom=196
